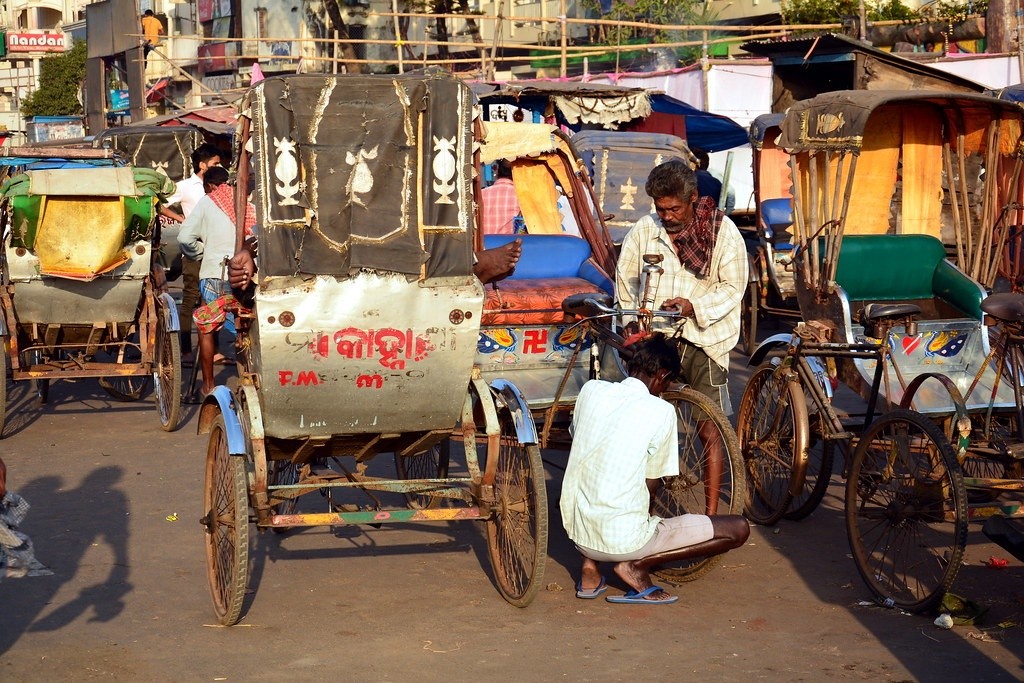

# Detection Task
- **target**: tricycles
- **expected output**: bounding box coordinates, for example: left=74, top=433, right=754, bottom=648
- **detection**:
left=742, top=114, right=790, bottom=341
left=749, top=90, right=1024, bottom=554
left=195, top=71, right=548, bottom=624
left=446, top=120, right=744, bottom=582
left=1, top=167, right=182, bottom=433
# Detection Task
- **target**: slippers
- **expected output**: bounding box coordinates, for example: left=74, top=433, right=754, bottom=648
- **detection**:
left=184, top=388, right=205, bottom=404
left=606, top=585, right=679, bottom=604
left=184, top=360, right=203, bottom=369
left=577, top=576, right=607, bottom=598
left=212, top=357, right=236, bottom=364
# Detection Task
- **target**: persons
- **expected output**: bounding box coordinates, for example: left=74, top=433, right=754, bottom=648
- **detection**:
left=558, top=331, right=750, bottom=605
left=177, top=167, right=259, bottom=404
left=142, top=9, right=164, bottom=46
left=159, top=142, right=240, bottom=370
left=480, top=163, right=522, bottom=234
left=614, top=160, right=750, bottom=517
left=694, top=152, right=722, bottom=206
left=227, top=223, right=522, bottom=285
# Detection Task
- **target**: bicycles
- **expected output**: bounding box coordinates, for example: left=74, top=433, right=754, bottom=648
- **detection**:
left=746, top=301, right=966, bottom=607
